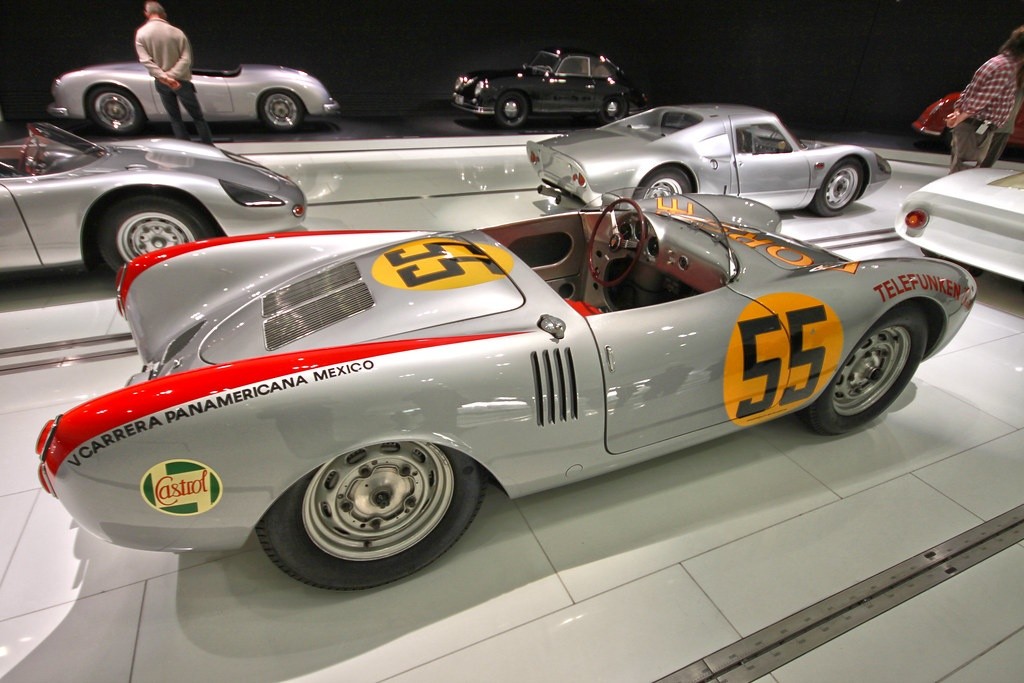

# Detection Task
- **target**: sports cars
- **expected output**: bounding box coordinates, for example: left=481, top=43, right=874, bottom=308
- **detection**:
left=892, top=165, right=1024, bottom=290
left=1, top=119, right=308, bottom=278
left=525, top=102, right=891, bottom=212
left=35, top=193, right=979, bottom=589
left=48, top=62, right=339, bottom=133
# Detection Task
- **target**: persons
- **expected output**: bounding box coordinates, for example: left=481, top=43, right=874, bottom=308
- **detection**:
left=134, top=1, right=215, bottom=146
left=943, top=25, right=1024, bottom=175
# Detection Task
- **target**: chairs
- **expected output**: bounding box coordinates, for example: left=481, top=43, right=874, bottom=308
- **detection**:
left=736, top=129, right=753, bottom=154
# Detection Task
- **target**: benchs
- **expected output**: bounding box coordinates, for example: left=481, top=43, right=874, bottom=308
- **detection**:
left=579, top=60, right=610, bottom=76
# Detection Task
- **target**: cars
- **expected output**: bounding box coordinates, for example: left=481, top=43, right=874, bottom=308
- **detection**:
left=450, top=47, right=672, bottom=128
left=912, top=91, right=1024, bottom=156
